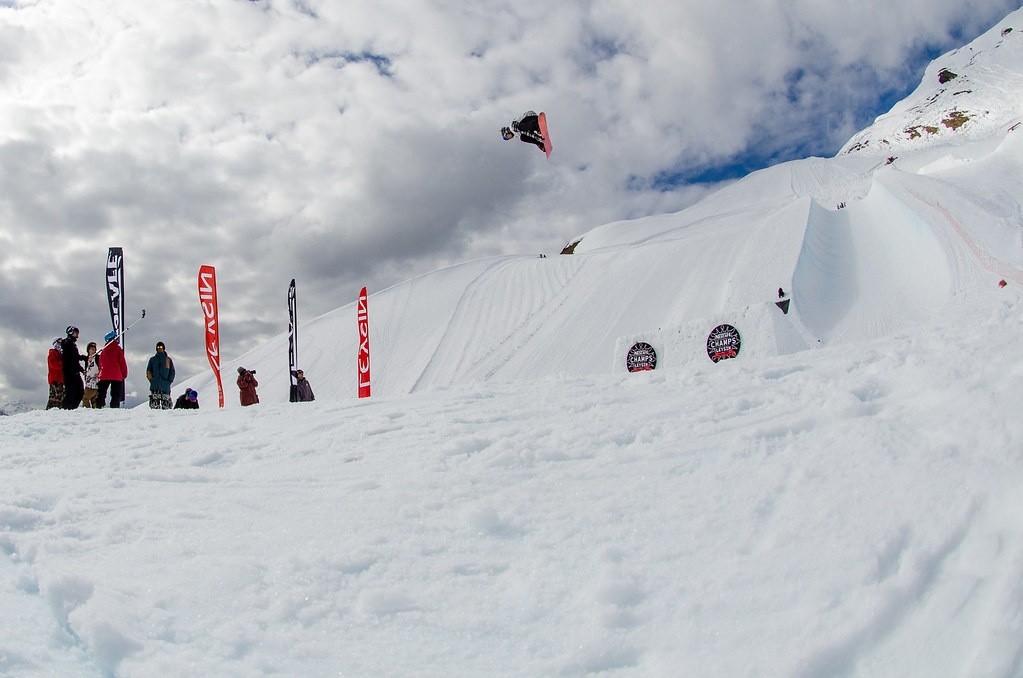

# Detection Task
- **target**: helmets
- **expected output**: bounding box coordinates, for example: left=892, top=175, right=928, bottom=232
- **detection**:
left=104, top=331, right=117, bottom=343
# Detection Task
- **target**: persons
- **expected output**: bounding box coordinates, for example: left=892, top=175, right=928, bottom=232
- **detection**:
left=95, top=330, right=127, bottom=408
left=174, top=389, right=199, bottom=410
left=236, top=367, right=259, bottom=406
left=500, top=110, right=547, bottom=152
left=81, top=341, right=102, bottom=409
left=61, top=326, right=93, bottom=409
left=296, top=369, right=315, bottom=402
left=46, top=337, right=65, bottom=409
left=146, top=342, right=175, bottom=410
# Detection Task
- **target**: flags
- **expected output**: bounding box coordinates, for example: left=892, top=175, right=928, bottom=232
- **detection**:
left=106, top=248, right=124, bottom=351
left=357, top=286, right=371, bottom=398
left=198, top=265, right=224, bottom=408
left=288, top=278, right=298, bottom=402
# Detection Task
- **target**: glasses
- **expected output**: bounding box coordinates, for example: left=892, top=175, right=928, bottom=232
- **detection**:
left=90, top=345, right=97, bottom=348
left=157, top=345, right=163, bottom=349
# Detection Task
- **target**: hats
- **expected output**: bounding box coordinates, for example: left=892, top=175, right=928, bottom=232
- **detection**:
left=238, top=367, right=245, bottom=374
left=188, top=390, right=197, bottom=402
left=156, top=342, right=165, bottom=351
left=52, top=337, right=63, bottom=350
left=66, top=326, right=75, bottom=336
left=501, top=127, right=511, bottom=140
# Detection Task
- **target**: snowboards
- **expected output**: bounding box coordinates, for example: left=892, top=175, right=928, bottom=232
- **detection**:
left=537, top=112, right=553, bottom=159
